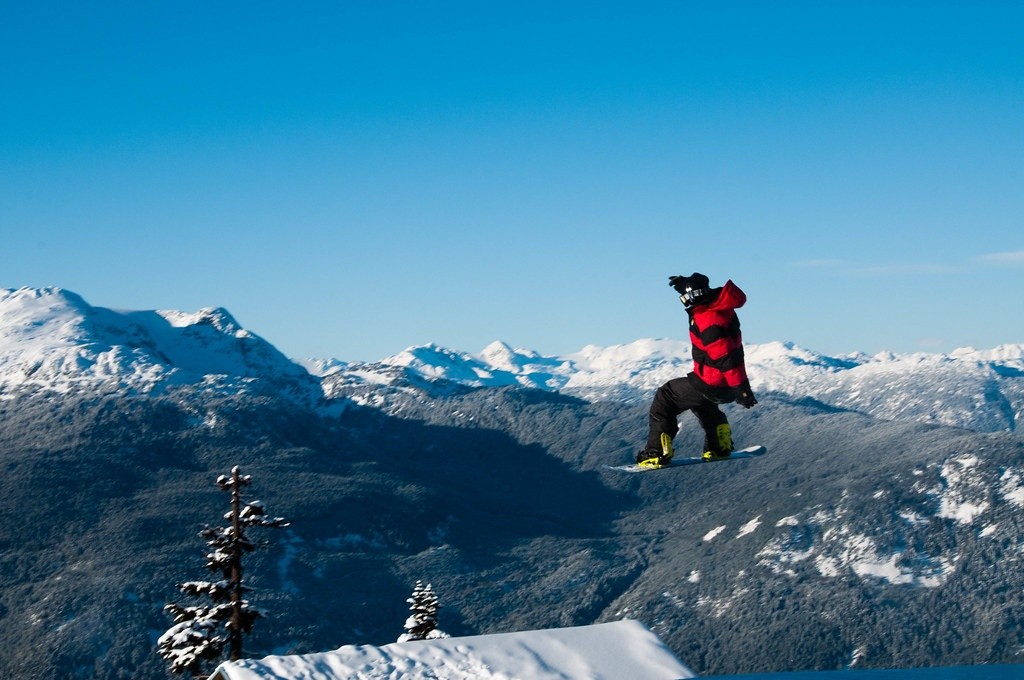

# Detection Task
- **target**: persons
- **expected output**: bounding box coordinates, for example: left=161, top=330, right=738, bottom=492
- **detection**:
left=630, top=271, right=757, bottom=467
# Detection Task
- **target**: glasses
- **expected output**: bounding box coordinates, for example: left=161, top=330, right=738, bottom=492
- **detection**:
left=678, top=294, right=689, bottom=307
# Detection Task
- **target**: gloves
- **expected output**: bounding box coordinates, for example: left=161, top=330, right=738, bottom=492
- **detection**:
left=668, top=275, right=686, bottom=287
left=735, top=389, right=758, bottom=409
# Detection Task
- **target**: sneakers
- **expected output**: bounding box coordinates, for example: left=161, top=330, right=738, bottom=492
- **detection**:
left=635, top=432, right=674, bottom=468
left=702, top=423, right=734, bottom=463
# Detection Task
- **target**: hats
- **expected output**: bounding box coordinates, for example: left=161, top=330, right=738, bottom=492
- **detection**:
left=677, top=273, right=709, bottom=302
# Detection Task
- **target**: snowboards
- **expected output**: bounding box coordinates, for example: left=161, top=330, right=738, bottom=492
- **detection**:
left=599, top=444, right=768, bottom=474
